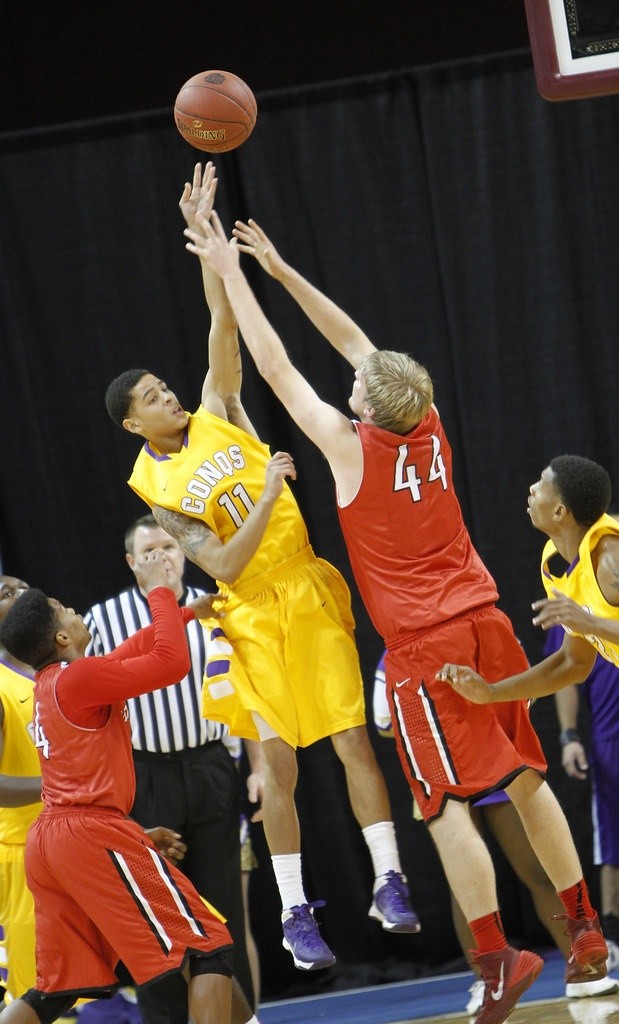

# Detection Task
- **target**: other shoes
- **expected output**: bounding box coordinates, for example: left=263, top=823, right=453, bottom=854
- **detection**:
left=466, top=980, right=486, bottom=1015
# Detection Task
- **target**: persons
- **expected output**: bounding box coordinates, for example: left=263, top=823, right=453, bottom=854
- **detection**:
left=183, top=210, right=619, bottom=1024
left=0, top=514, right=265, bottom=1024
left=373, top=455, right=619, bottom=1015
left=105, top=161, right=422, bottom=970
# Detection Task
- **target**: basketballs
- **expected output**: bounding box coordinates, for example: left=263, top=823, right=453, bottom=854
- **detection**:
left=173, top=69, right=258, bottom=154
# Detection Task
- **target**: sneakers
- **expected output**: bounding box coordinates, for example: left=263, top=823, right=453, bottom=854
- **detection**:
left=548, top=906, right=609, bottom=966
left=468, top=944, right=545, bottom=1024
left=282, top=899, right=335, bottom=971
left=564, top=944, right=619, bottom=997
left=369, top=869, right=420, bottom=932
